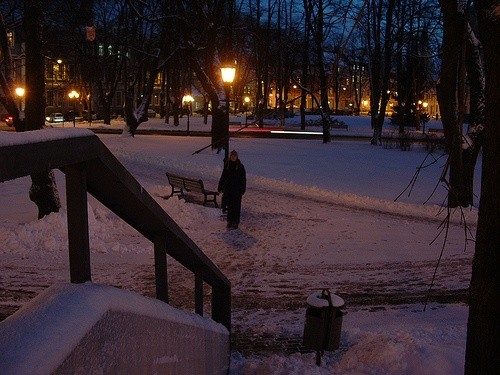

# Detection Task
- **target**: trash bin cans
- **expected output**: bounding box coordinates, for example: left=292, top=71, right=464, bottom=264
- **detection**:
left=302, top=288, right=345, bottom=352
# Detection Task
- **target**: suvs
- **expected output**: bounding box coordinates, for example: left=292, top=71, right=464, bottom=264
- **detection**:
left=273, top=108, right=295, bottom=119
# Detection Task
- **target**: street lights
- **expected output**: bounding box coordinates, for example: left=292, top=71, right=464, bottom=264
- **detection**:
left=244, top=96, right=251, bottom=127
left=182, top=95, right=195, bottom=133
left=16, top=88, right=25, bottom=113
left=68, top=91, right=80, bottom=127
left=219, top=50, right=239, bottom=170
left=293, top=85, right=297, bottom=108
left=417, top=99, right=429, bottom=111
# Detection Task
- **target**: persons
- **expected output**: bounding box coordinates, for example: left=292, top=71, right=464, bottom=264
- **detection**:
left=218, top=149, right=247, bottom=232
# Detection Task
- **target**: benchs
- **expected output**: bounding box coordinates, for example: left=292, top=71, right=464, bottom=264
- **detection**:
left=164, top=172, right=220, bottom=208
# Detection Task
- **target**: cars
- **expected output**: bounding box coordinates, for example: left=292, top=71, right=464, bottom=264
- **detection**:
left=147, top=108, right=156, bottom=118
left=310, top=108, right=320, bottom=112
left=49, top=113, right=65, bottom=123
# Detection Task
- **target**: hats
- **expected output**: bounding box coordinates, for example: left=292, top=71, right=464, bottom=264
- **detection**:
left=230, top=150, right=238, bottom=157
